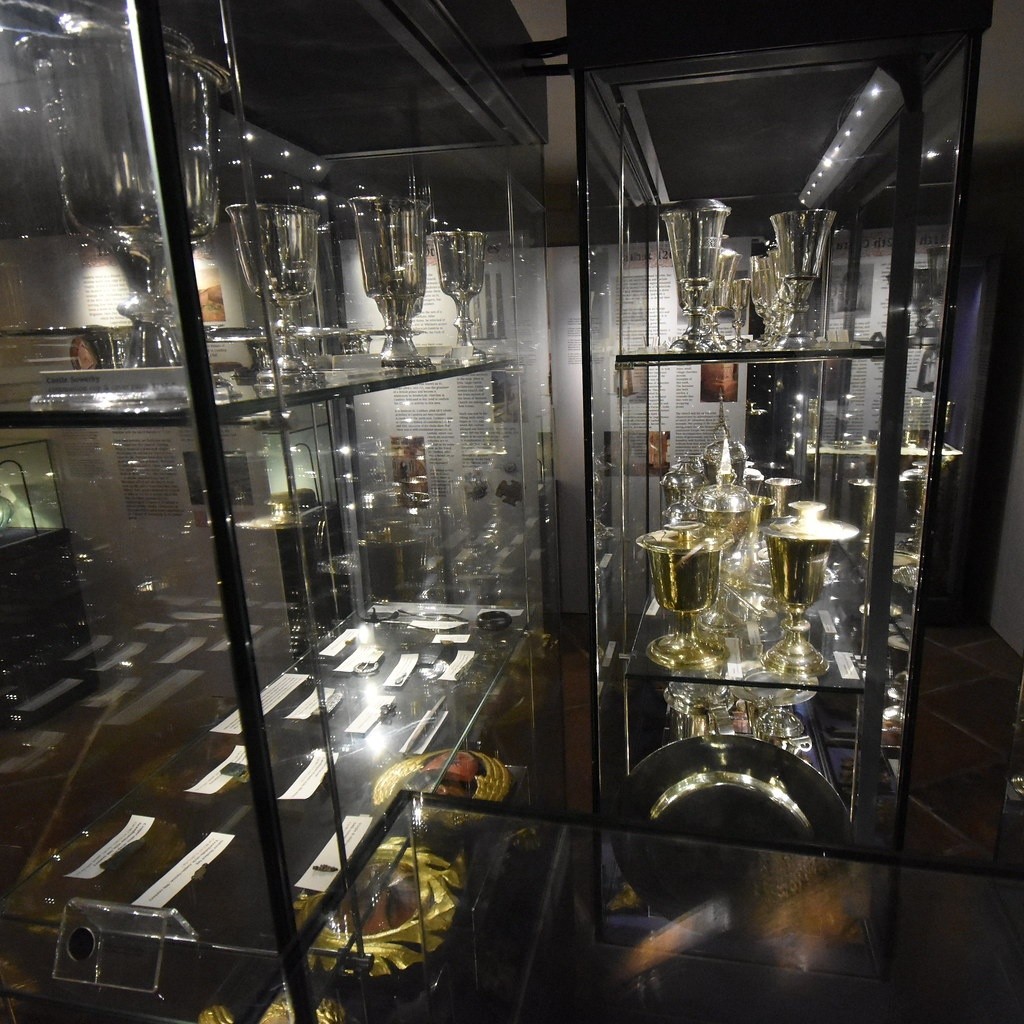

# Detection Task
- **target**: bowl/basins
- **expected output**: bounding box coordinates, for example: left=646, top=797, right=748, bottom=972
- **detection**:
left=476, top=611, right=512, bottom=630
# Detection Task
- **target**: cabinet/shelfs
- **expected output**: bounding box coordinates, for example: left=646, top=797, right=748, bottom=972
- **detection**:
left=0, top=0, right=1024, bottom=1024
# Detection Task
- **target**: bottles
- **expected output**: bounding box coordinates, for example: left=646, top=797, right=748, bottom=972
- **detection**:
left=87, top=327, right=125, bottom=369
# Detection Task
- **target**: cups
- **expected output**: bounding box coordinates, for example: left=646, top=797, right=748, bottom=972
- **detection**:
left=908, top=396, right=923, bottom=442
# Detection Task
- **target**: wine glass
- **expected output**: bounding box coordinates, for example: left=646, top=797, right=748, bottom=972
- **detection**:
left=347, top=196, right=436, bottom=368
left=750, top=210, right=836, bottom=349
left=744, top=462, right=802, bottom=564
left=918, top=399, right=954, bottom=447
left=14, top=36, right=242, bottom=400
left=430, top=231, right=488, bottom=365
left=226, top=205, right=323, bottom=387
left=728, top=278, right=750, bottom=353
left=848, top=480, right=876, bottom=543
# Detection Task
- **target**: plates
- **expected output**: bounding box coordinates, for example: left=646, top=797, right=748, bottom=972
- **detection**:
left=863, top=550, right=919, bottom=566
left=732, top=668, right=819, bottom=706
left=609, top=734, right=850, bottom=921
